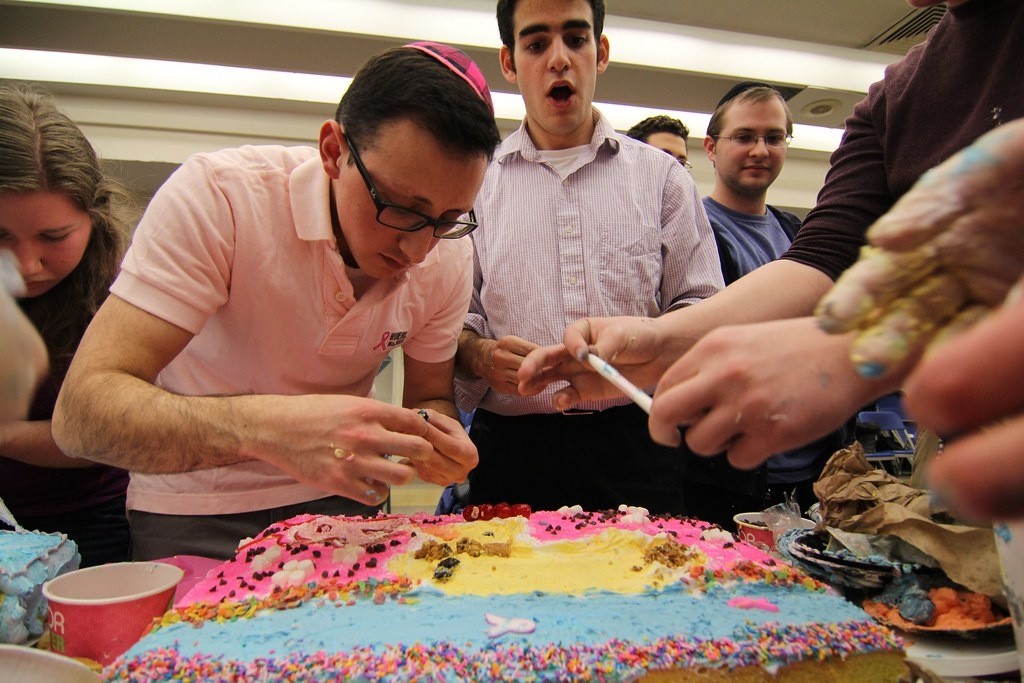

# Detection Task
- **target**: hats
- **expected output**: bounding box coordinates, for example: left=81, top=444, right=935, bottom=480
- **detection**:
left=403, top=42, right=495, bottom=116
left=716, top=80, right=772, bottom=107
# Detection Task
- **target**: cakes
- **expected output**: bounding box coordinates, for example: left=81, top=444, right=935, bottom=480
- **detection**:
left=0, top=525, right=82, bottom=646
left=93, top=502, right=913, bottom=683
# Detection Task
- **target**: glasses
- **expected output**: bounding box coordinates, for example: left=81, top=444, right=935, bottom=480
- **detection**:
left=679, top=160, right=692, bottom=173
left=341, top=133, right=477, bottom=239
left=710, top=133, right=795, bottom=148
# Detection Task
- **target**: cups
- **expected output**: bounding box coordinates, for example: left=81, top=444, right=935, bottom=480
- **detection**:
left=733, top=512, right=817, bottom=555
left=41, top=562, right=185, bottom=669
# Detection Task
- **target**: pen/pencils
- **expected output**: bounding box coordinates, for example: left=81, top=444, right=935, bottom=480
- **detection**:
left=587, top=353, right=652, bottom=416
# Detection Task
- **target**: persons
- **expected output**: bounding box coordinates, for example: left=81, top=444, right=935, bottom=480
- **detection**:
left=450, top=0, right=727, bottom=516
left=625, top=0, right=1023, bottom=525
left=0, top=80, right=142, bottom=569
left=53, top=42, right=504, bottom=560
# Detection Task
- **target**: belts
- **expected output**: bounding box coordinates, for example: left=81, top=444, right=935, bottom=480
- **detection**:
left=543, top=407, right=598, bottom=416
